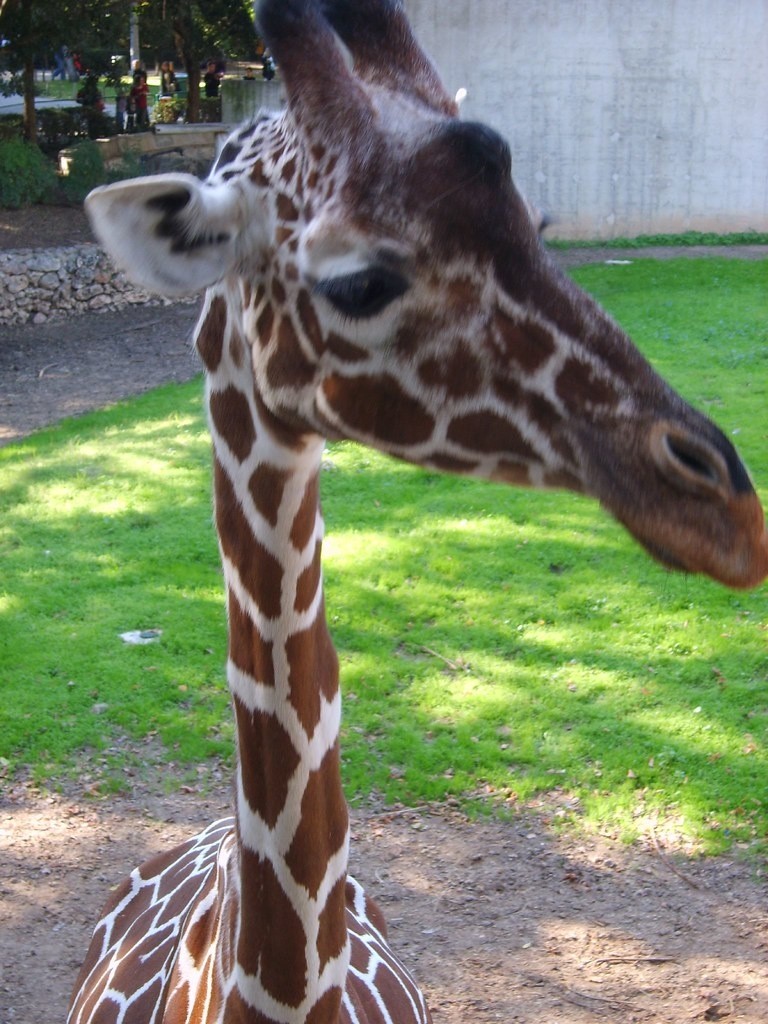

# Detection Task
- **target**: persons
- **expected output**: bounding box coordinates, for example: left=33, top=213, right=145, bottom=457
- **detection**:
left=116, top=62, right=150, bottom=132
left=262, top=48, right=275, bottom=82
left=242, top=68, right=255, bottom=81
left=52, top=45, right=82, bottom=81
left=77, top=76, right=105, bottom=140
left=161, top=62, right=180, bottom=97
left=204, top=61, right=220, bottom=98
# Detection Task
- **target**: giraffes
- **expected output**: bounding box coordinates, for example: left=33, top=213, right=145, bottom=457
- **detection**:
left=64, top=1, right=767, bottom=1024
